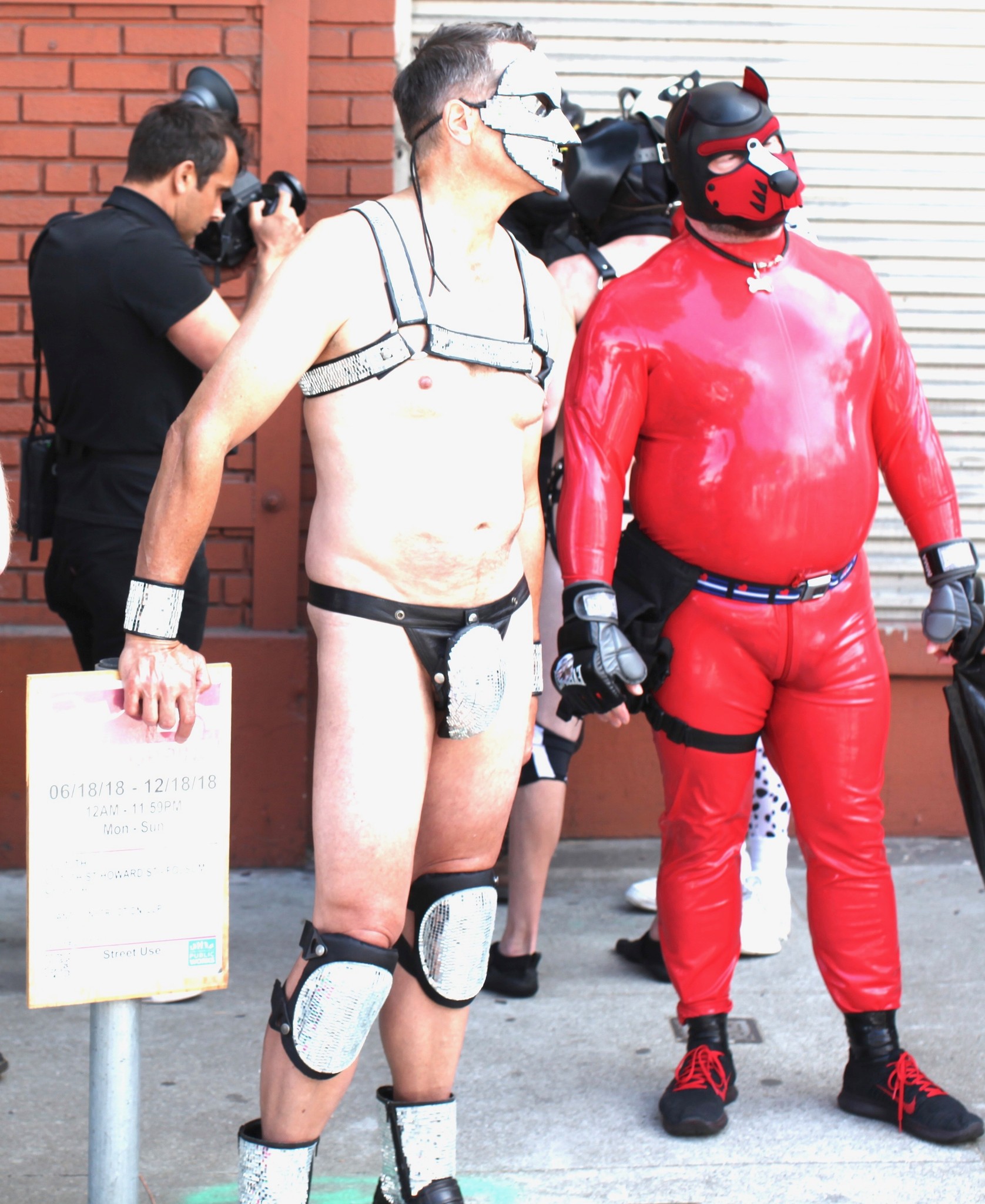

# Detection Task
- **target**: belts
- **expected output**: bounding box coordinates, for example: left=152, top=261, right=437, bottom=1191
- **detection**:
left=693, top=555, right=860, bottom=608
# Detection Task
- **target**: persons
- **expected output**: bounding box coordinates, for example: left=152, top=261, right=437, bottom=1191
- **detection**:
left=0, top=461, right=11, bottom=1077
left=27, top=99, right=302, bottom=1009
left=479, top=112, right=796, bottom=1000
left=555, top=63, right=985, bottom=1148
left=117, top=18, right=578, bottom=1204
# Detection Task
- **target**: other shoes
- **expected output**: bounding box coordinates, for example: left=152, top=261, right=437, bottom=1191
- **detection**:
left=483, top=942, right=542, bottom=998
left=615, top=930, right=677, bottom=982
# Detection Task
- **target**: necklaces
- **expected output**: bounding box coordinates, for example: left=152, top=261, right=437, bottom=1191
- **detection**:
left=683, top=220, right=790, bottom=294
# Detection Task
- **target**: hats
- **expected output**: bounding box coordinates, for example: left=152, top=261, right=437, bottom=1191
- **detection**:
left=661, top=66, right=806, bottom=233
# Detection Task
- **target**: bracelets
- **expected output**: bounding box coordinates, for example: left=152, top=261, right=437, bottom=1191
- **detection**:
left=124, top=572, right=187, bottom=640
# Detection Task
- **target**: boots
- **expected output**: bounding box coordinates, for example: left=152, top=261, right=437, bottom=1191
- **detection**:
left=234, top=1120, right=322, bottom=1204
left=369, top=1085, right=468, bottom=1204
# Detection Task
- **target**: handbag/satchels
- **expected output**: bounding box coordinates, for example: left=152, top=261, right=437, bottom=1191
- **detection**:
left=18, top=430, right=56, bottom=561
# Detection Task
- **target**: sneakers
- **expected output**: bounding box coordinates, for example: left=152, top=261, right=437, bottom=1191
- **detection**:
left=658, top=1039, right=739, bottom=1137
left=741, top=869, right=792, bottom=955
left=838, top=1047, right=984, bottom=1145
left=625, top=876, right=657, bottom=912
